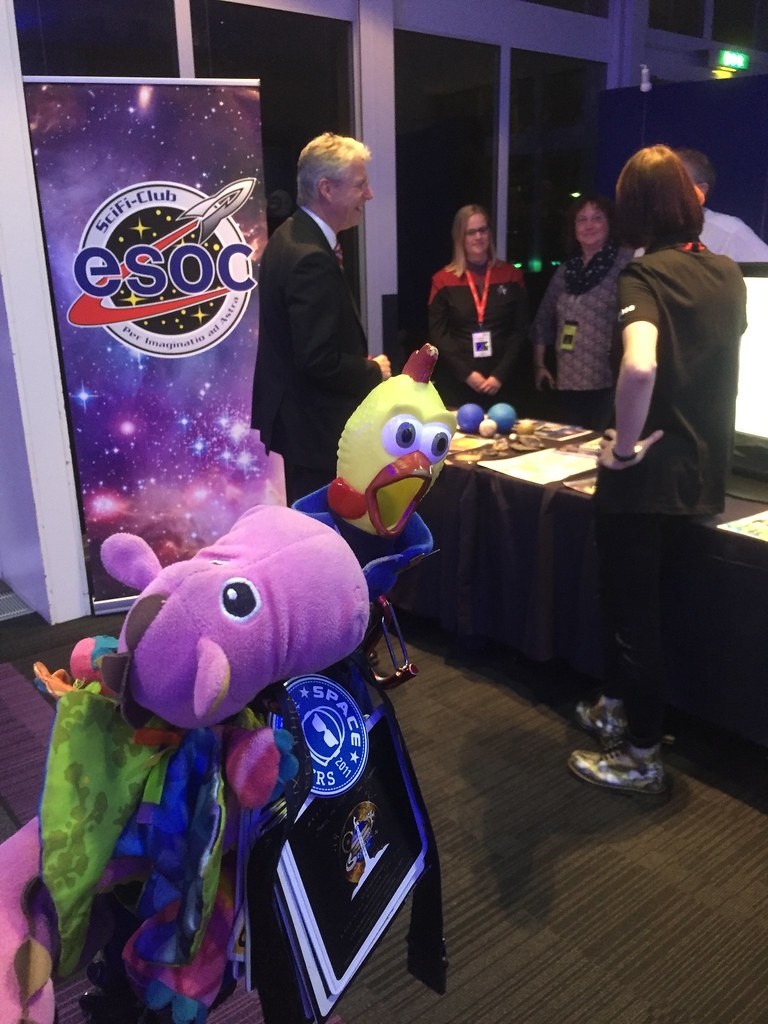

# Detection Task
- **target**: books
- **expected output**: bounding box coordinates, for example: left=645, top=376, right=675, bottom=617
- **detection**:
left=511, top=416, right=605, bottom=457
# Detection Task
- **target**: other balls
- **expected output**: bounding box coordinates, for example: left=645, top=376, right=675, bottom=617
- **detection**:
left=479, top=420, right=497, bottom=437
left=517, top=421, right=534, bottom=436
left=457, top=403, right=483, bottom=432
left=488, top=403, right=515, bottom=431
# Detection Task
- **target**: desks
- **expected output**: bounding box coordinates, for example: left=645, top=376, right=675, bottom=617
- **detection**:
left=379, top=428, right=768, bottom=750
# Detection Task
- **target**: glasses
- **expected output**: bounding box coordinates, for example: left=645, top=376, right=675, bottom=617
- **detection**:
left=465, top=226, right=488, bottom=236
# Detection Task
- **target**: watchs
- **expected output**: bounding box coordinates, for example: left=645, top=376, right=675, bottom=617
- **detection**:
left=611, top=445, right=637, bottom=461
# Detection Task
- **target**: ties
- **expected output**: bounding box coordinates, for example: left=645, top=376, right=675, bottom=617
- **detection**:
left=333, top=239, right=344, bottom=272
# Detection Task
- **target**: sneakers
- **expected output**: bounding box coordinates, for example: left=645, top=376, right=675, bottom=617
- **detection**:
left=567, top=742, right=669, bottom=797
left=575, top=703, right=626, bottom=744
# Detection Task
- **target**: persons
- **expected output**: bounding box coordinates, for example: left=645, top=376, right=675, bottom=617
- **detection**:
left=530, top=193, right=636, bottom=435
left=426, top=202, right=537, bottom=409
left=249, top=132, right=392, bottom=509
left=566, top=140, right=749, bottom=797
left=627, top=151, right=768, bottom=263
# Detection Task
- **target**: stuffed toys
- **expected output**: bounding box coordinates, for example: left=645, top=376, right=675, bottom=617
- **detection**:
left=0, top=503, right=376, bottom=1023
